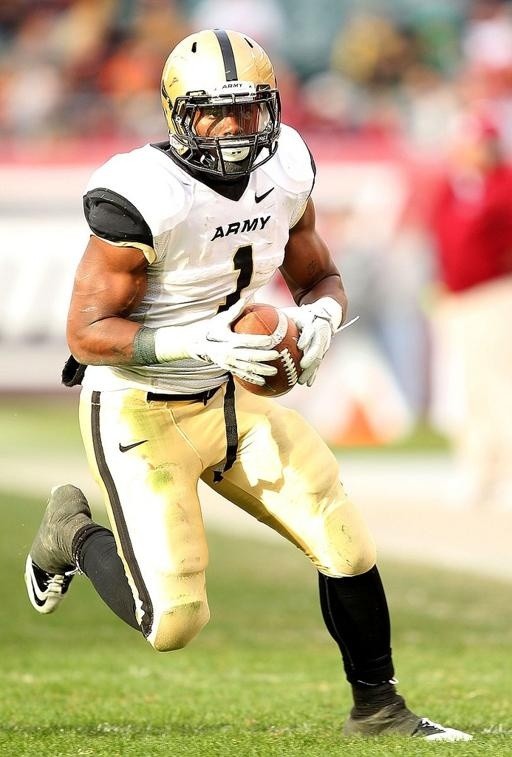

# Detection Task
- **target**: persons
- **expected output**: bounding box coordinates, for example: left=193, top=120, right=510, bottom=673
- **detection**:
left=1, top=1, right=512, bottom=508
left=12, top=26, right=477, bottom=745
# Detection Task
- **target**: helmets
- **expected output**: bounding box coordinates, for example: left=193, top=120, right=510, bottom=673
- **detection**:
left=160, top=30, right=281, bottom=179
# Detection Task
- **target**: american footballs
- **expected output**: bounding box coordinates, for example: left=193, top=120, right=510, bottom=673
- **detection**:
left=230, top=305, right=303, bottom=398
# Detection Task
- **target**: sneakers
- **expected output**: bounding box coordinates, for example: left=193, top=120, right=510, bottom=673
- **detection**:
left=24, top=483, right=91, bottom=613
left=345, top=695, right=473, bottom=742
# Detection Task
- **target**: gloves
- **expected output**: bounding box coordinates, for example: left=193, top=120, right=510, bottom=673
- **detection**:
left=279, top=296, right=359, bottom=387
left=155, top=294, right=280, bottom=386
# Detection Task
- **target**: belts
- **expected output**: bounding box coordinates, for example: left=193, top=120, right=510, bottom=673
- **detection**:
left=147, top=386, right=221, bottom=401
